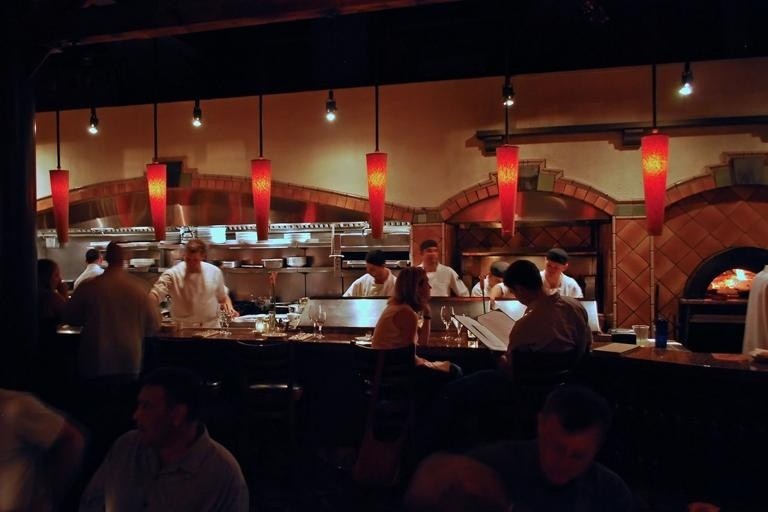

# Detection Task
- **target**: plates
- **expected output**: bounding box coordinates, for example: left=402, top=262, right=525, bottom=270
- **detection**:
left=354, top=336, right=373, bottom=341
left=711, top=353, right=752, bottom=362
left=129, top=259, right=155, bottom=266
left=165, top=224, right=311, bottom=243
left=286, top=256, right=307, bottom=267
left=260, top=333, right=287, bottom=338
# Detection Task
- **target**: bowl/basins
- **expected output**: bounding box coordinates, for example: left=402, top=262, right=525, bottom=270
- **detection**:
left=610, top=333, right=636, bottom=344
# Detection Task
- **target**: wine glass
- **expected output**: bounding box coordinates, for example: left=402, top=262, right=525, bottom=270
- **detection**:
left=308, top=303, right=320, bottom=337
left=440, top=305, right=453, bottom=339
left=216, top=303, right=233, bottom=335
left=314, top=310, right=326, bottom=339
left=452, top=313, right=465, bottom=341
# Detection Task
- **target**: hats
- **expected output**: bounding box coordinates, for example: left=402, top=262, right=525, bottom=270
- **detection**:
left=365, top=249, right=387, bottom=266
left=489, top=260, right=510, bottom=278
left=545, top=247, right=569, bottom=265
left=421, top=240, right=439, bottom=249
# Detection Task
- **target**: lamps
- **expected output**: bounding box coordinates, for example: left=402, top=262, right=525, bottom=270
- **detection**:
left=190, top=100, right=203, bottom=127
left=145, top=105, right=170, bottom=242
left=87, top=106, right=99, bottom=135
left=501, top=76, right=516, bottom=108
left=365, top=86, right=390, bottom=239
left=324, top=90, right=339, bottom=123
left=676, top=61, right=697, bottom=97
left=496, top=109, right=521, bottom=237
left=250, top=95, right=272, bottom=242
left=47, top=110, right=72, bottom=250
left=640, top=65, right=670, bottom=238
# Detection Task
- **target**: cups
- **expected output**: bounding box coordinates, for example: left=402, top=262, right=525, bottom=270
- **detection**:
left=467, top=341, right=478, bottom=348
left=176, top=319, right=183, bottom=330
left=632, top=324, right=649, bottom=346
left=466, top=329, right=478, bottom=341
left=652, top=319, right=668, bottom=349
left=191, top=320, right=203, bottom=336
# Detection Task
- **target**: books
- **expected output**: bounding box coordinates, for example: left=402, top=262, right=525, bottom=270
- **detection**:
left=593, top=342, right=640, bottom=354
left=450, top=308, right=517, bottom=352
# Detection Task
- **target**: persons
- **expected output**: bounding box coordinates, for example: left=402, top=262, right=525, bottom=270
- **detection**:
left=339, top=250, right=397, bottom=299
left=416, top=240, right=470, bottom=297
left=371, top=266, right=467, bottom=399
left=72, top=248, right=105, bottom=291
left=145, top=239, right=241, bottom=328
left=38, top=258, right=69, bottom=323
left=464, top=383, right=638, bottom=512
left=494, top=259, right=592, bottom=389
left=488, top=247, right=584, bottom=310
left=67, top=238, right=164, bottom=378
left=470, top=259, right=511, bottom=296
left=74, top=358, right=252, bottom=512
left=0, top=385, right=80, bottom=510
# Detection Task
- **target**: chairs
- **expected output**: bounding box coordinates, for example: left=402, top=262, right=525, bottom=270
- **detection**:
left=158, top=338, right=222, bottom=424
left=347, top=338, right=424, bottom=441
left=235, top=333, right=307, bottom=476
left=509, top=343, right=590, bottom=441
